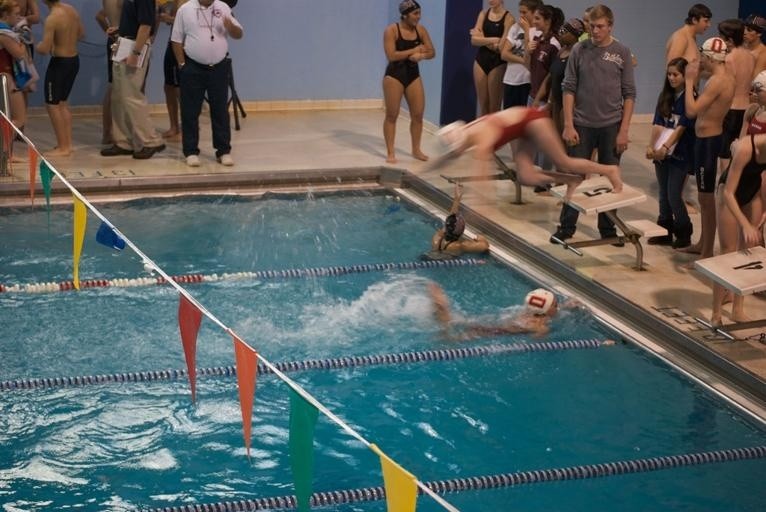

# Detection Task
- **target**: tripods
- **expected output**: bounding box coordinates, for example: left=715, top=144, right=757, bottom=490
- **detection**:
left=198, top=58, right=246, bottom=130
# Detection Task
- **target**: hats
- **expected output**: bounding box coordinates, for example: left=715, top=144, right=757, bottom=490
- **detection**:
left=699, top=37, right=727, bottom=62
left=524, top=288, right=553, bottom=313
left=399, top=0, right=421, bottom=15
left=445, top=213, right=465, bottom=236
left=435, top=126, right=464, bottom=153
left=752, top=72, right=766, bottom=91
left=564, top=17, right=585, bottom=38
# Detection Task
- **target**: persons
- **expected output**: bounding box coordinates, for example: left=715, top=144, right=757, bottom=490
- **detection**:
left=421, top=106, right=622, bottom=202
left=1, top=0, right=84, bottom=164
left=94, top=0, right=242, bottom=166
left=470, top=2, right=636, bottom=247
left=648, top=3, right=766, bottom=330
left=426, top=182, right=488, bottom=259
left=383, top=0, right=436, bottom=163
left=427, top=282, right=577, bottom=340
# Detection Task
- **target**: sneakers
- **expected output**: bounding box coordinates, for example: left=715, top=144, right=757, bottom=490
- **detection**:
left=648, top=234, right=690, bottom=250
left=135, top=144, right=165, bottom=160
left=216, top=154, right=233, bottom=165
left=550, top=229, right=571, bottom=244
left=186, top=154, right=199, bottom=167
left=101, top=145, right=134, bottom=156
left=599, top=227, right=623, bottom=246
